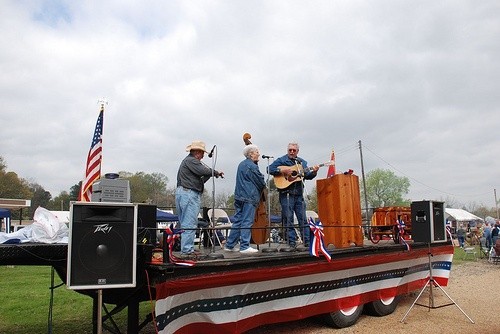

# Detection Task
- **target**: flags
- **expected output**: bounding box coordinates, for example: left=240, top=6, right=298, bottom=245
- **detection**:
left=77, top=109, right=104, bottom=203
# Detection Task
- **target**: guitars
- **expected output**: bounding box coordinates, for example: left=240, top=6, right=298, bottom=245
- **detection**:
left=274, top=159, right=335, bottom=190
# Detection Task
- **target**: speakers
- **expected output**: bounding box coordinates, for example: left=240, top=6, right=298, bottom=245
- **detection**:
left=411, top=200, right=448, bottom=243
left=66, top=201, right=138, bottom=289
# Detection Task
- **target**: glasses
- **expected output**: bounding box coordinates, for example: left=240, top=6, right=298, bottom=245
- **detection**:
left=288, top=149, right=298, bottom=153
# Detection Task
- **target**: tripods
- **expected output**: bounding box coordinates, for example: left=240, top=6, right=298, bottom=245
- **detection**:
left=400, top=243, right=475, bottom=324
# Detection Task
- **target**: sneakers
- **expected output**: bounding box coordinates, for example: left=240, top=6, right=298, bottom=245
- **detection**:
left=239, top=247, right=259, bottom=253
left=305, top=240, right=313, bottom=246
left=224, top=245, right=239, bottom=252
left=290, top=242, right=296, bottom=248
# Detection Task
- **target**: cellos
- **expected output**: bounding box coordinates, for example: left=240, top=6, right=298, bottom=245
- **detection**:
left=243, top=133, right=270, bottom=245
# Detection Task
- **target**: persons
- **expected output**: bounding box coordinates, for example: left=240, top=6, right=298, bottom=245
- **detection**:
left=266, top=141, right=320, bottom=249
left=174, top=141, right=224, bottom=256
left=223, top=144, right=266, bottom=253
left=458, top=219, right=500, bottom=252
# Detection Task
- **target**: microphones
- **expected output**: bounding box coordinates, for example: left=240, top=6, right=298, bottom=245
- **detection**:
left=262, top=155, right=273, bottom=159
left=208, top=145, right=216, bottom=158
left=288, top=156, right=299, bottom=161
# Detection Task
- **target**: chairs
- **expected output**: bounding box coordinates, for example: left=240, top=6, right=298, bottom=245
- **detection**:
left=461, top=240, right=478, bottom=261
left=479, top=238, right=489, bottom=259
left=491, top=244, right=500, bottom=266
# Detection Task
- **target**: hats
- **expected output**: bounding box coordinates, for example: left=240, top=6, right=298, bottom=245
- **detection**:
left=185, top=141, right=209, bottom=153
